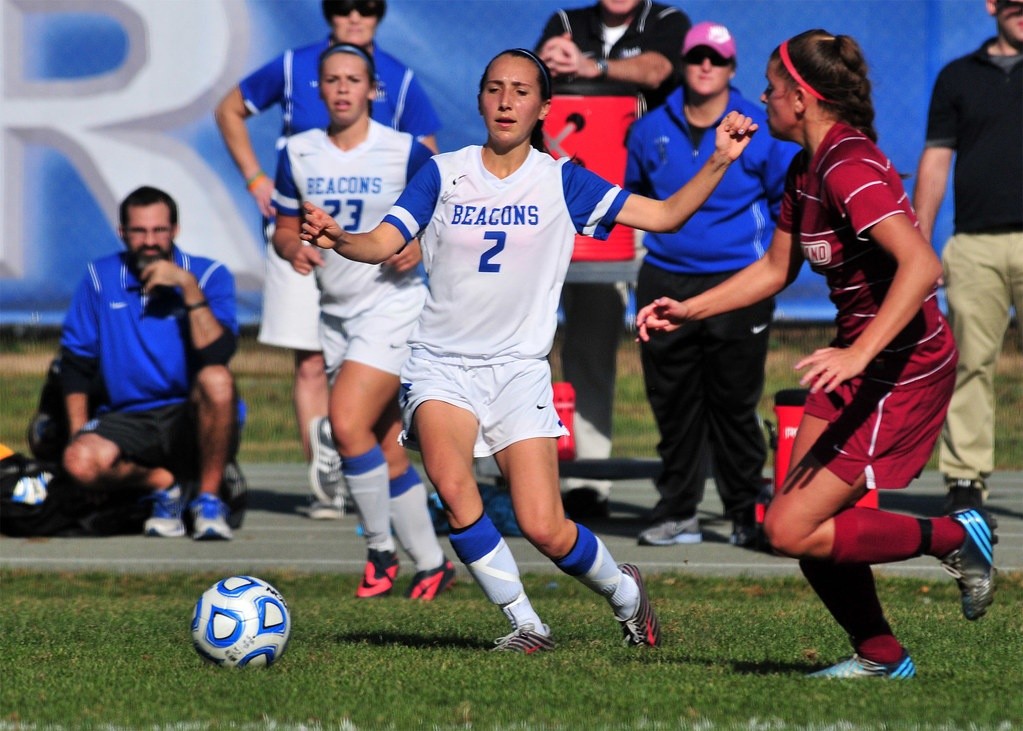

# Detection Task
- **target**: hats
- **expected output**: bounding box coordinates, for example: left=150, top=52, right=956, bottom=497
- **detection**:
left=682, top=20, right=737, bottom=61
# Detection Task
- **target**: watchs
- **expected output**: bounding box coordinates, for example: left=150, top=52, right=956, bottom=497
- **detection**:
left=597, top=57, right=608, bottom=80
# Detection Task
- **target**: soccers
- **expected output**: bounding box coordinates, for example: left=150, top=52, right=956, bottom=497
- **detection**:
left=189, top=573, right=293, bottom=674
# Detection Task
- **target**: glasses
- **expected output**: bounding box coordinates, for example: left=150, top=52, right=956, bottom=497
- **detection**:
left=331, top=3, right=381, bottom=17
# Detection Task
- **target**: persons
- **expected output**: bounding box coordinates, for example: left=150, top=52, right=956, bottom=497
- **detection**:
left=272, top=42, right=458, bottom=600
left=61, top=185, right=245, bottom=541
left=634, top=27, right=998, bottom=679
left=299, top=47, right=758, bottom=656
left=215, top=0, right=443, bottom=516
left=531, top=0, right=693, bottom=522
left=914, top=0, right=1023, bottom=511
left=624, top=22, right=800, bottom=543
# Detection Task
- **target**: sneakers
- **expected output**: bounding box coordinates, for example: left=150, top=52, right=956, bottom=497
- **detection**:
left=307, top=416, right=347, bottom=519
left=637, top=516, right=703, bottom=546
left=144, top=482, right=190, bottom=538
left=613, top=564, right=659, bottom=647
left=806, top=649, right=918, bottom=679
left=942, top=510, right=999, bottom=620
left=492, top=623, right=556, bottom=655
left=189, top=495, right=233, bottom=543
left=355, top=549, right=399, bottom=598
left=404, top=556, right=459, bottom=600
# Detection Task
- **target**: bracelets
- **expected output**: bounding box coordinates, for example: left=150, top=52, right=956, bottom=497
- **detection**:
left=185, top=300, right=209, bottom=311
left=248, top=170, right=264, bottom=191
